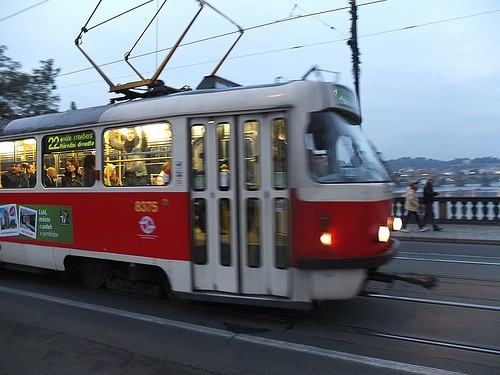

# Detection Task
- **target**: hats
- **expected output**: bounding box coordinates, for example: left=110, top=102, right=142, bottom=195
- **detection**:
left=408, top=180, right=419, bottom=186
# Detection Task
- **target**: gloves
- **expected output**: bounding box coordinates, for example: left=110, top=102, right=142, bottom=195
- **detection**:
left=433, top=192, right=440, bottom=196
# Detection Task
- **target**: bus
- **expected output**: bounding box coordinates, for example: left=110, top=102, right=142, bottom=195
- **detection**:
left=0, top=1, right=402, bottom=318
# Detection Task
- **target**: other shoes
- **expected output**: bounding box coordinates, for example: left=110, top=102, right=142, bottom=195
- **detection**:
left=401, top=229, right=412, bottom=233
left=419, top=227, right=429, bottom=232
left=433, top=226, right=442, bottom=231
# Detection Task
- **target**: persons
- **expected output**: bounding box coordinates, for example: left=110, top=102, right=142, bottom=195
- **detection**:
left=195, top=198, right=228, bottom=235
left=24, top=165, right=37, bottom=188
left=1, top=163, right=26, bottom=188
left=420, top=177, right=443, bottom=231
left=62, top=157, right=84, bottom=187
left=307, top=148, right=322, bottom=181
left=103, top=164, right=121, bottom=186
left=400, top=180, right=430, bottom=232
left=82, top=154, right=96, bottom=187
left=157, top=162, right=171, bottom=186
left=44, top=167, right=61, bottom=187
left=110, top=126, right=151, bottom=186
